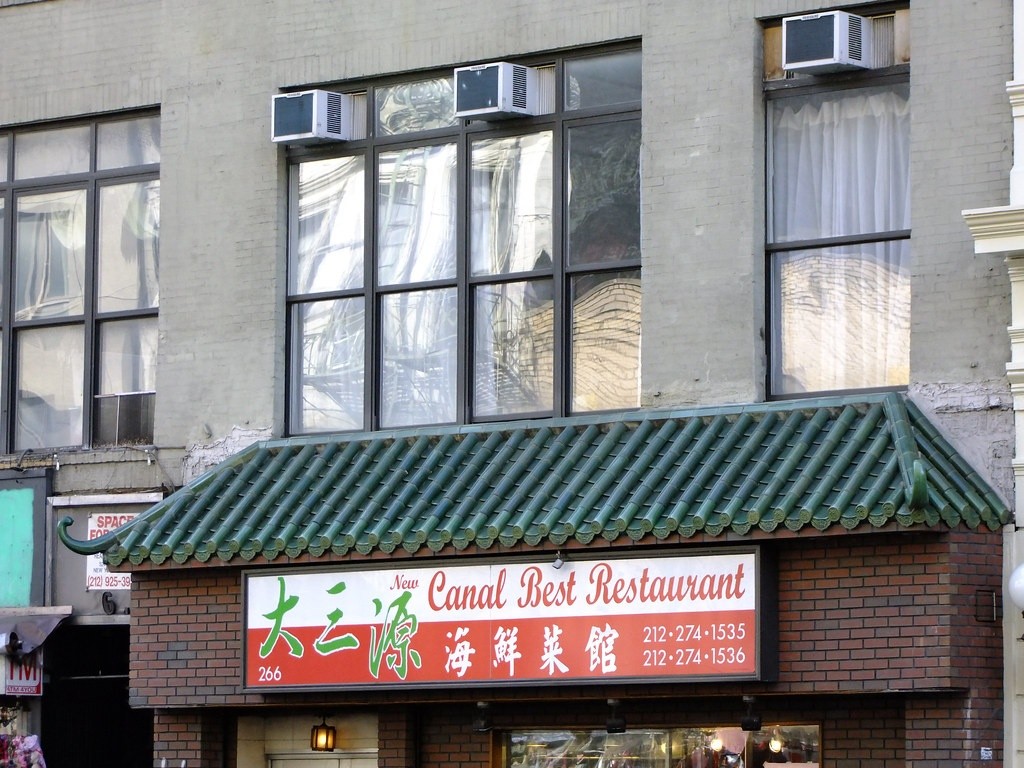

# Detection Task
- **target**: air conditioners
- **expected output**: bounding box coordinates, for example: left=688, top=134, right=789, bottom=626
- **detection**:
left=270, top=88, right=351, bottom=143
left=454, top=61, right=540, bottom=120
left=780, top=10, right=871, bottom=74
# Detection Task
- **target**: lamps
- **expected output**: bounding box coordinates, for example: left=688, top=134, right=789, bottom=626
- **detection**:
left=739, top=696, right=762, bottom=731
left=310, top=718, right=339, bottom=753
left=471, top=702, right=494, bottom=732
left=606, top=699, right=626, bottom=733
